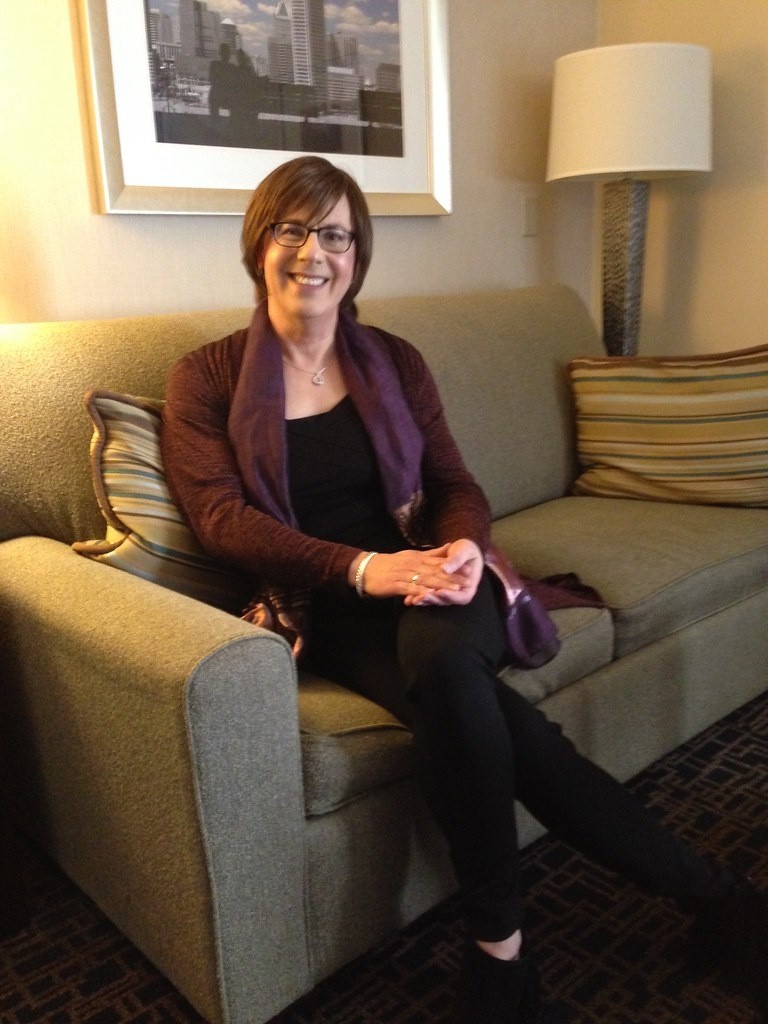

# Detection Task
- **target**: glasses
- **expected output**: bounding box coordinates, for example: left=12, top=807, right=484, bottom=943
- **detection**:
left=268, top=221, right=357, bottom=253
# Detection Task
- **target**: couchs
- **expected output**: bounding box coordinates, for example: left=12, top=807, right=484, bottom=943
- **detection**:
left=0, top=285, right=768, bottom=1024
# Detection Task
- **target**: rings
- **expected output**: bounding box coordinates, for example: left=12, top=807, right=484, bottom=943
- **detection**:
left=412, top=575, right=419, bottom=584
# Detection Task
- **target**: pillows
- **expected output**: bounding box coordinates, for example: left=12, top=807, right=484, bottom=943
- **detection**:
left=66, top=387, right=254, bottom=617
left=565, top=343, right=768, bottom=509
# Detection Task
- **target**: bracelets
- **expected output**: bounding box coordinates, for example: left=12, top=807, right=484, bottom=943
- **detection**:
left=355, top=551, right=378, bottom=598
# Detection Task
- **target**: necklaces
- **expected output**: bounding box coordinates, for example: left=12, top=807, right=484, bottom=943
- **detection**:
left=282, top=352, right=337, bottom=384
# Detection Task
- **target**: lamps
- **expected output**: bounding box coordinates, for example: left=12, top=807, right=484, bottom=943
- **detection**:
left=542, top=41, right=713, bottom=355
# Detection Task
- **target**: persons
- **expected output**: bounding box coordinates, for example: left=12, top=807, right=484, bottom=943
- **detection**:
left=208, top=44, right=259, bottom=119
left=161, top=156, right=768, bottom=1024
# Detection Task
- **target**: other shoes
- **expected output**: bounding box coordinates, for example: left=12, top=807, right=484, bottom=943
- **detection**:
left=688, top=857, right=768, bottom=990
left=461, top=937, right=541, bottom=1024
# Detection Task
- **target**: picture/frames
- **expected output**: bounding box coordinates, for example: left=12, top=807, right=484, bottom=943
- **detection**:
left=75, top=0, right=453, bottom=217
